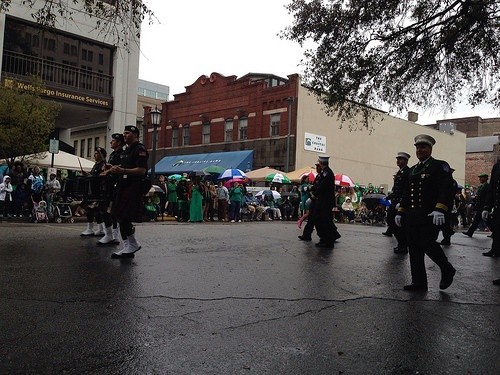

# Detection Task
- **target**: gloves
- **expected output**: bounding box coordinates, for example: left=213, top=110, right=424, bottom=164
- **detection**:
left=306, top=198, right=311, bottom=205
left=395, top=203, right=400, bottom=209
left=482, top=211, right=489, bottom=221
left=395, top=215, right=402, bottom=226
left=427, top=211, right=445, bottom=225
left=304, top=187, right=308, bottom=191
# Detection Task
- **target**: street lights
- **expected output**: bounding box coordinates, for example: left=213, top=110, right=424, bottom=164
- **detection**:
left=150, top=105, right=162, bottom=187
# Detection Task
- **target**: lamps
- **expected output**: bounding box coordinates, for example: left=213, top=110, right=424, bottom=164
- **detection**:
left=198, top=114, right=208, bottom=120
left=236, top=108, right=246, bottom=114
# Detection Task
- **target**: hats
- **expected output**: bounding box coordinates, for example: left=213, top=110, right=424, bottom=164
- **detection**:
left=318, top=155, right=330, bottom=163
left=111, top=133, right=126, bottom=145
left=169, top=178, right=177, bottom=182
left=94, top=147, right=106, bottom=156
left=478, top=174, right=488, bottom=177
left=414, top=134, right=436, bottom=148
left=395, top=152, right=410, bottom=161
left=315, top=161, right=321, bottom=165
left=124, top=125, right=140, bottom=137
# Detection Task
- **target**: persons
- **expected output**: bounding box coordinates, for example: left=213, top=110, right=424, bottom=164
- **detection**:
left=0, top=166, right=67, bottom=223
left=149, top=174, right=310, bottom=223
left=333, top=182, right=391, bottom=225
left=382, top=135, right=456, bottom=293
left=81, top=125, right=151, bottom=257
left=297, top=156, right=341, bottom=248
left=453, top=159, right=500, bottom=258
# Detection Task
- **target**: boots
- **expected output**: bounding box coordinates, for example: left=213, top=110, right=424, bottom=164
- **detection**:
left=81, top=222, right=141, bottom=256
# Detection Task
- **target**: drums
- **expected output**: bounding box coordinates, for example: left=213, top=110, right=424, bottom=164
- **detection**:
left=67, top=174, right=108, bottom=203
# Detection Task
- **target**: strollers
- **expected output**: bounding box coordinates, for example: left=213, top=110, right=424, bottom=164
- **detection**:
left=31, top=194, right=49, bottom=223
left=49, top=193, right=75, bottom=224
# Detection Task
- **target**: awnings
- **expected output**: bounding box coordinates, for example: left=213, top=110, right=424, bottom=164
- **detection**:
left=148, top=150, right=254, bottom=173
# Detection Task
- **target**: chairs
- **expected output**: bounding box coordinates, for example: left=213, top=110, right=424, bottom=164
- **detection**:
left=338, top=206, right=347, bottom=221
left=239, top=207, right=293, bottom=222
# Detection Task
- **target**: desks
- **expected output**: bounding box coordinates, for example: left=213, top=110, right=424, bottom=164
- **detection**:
left=165, top=200, right=187, bottom=222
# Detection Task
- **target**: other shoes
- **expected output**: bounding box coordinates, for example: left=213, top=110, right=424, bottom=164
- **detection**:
left=382, top=232, right=456, bottom=292
left=487, top=235, right=492, bottom=237
left=462, top=232, right=472, bottom=238
left=493, top=278, right=500, bottom=285
left=298, top=221, right=342, bottom=248
left=482, top=251, right=493, bottom=257
left=201, top=214, right=292, bottom=223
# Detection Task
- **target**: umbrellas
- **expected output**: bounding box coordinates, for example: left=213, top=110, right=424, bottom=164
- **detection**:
left=285, top=165, right=318, bottom=183
left=264, top=173, right=291, bottom=186
left=0, top=150, right=97, bottom=174
left=217, top=166, right=248, bottom=179
left=245, top=166, right=290, bottom=187
left=200, top=164, right=226, bottom=177
left=333, top=174, right=354, bottom=187
left=168, top=174, right=182, bottom=180
left=224, top=178, right=243, bottom=188
left=299, top=170, right=317, bottom=182
left=145, top=185, right=165, bottom=196
left=362, top=193, right=386, bottom=210
